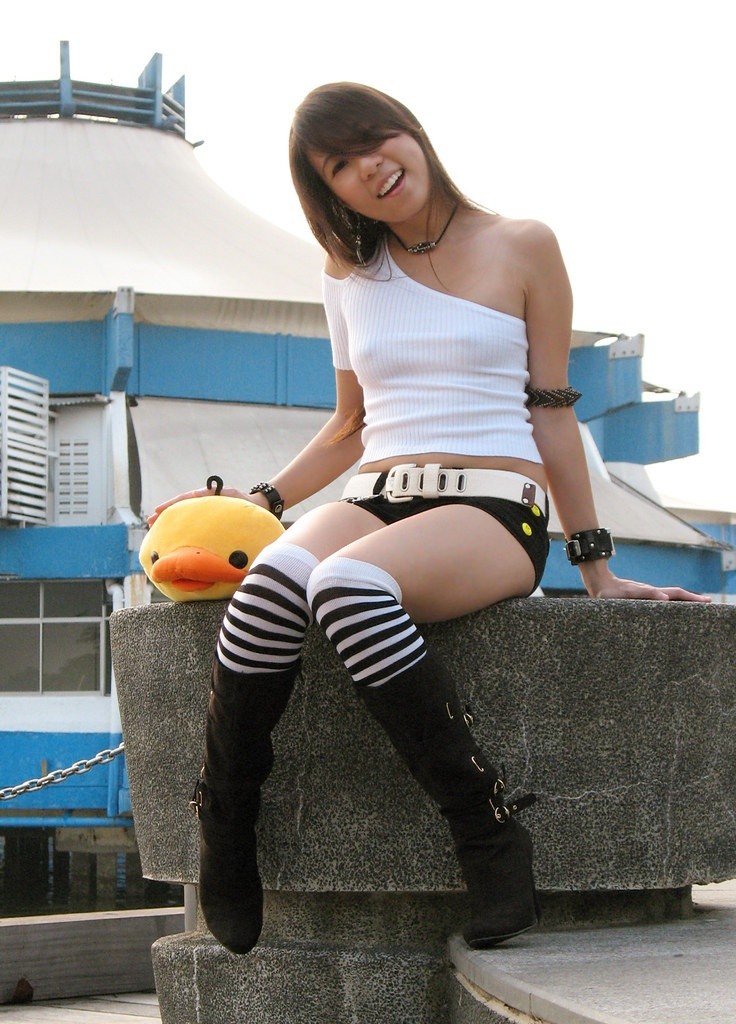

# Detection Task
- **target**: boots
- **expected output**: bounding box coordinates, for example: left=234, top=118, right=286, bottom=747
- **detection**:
left=353, top=653, right=542, bottom=945
left=190, top=651, right=300, bottom=956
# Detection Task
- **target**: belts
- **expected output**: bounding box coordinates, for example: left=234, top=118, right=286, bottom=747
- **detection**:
left=340, top=464, right=546, bottom=518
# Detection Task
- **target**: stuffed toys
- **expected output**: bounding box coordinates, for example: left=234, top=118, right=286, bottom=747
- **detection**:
left=139, top=495, right=286, bottom=602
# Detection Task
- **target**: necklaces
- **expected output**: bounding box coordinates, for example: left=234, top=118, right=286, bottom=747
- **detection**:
left=389, top=203, right=459, bottom=254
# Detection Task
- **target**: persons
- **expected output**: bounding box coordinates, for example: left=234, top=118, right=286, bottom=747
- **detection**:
left=145, top=83, right=714, bottom=954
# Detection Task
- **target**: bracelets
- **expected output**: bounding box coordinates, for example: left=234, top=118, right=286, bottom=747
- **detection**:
left=525, top=385, right=583, bottom=409
left=249, top=482, right=284, bottom=521
left=565, top=528, right=617, bottom=567
